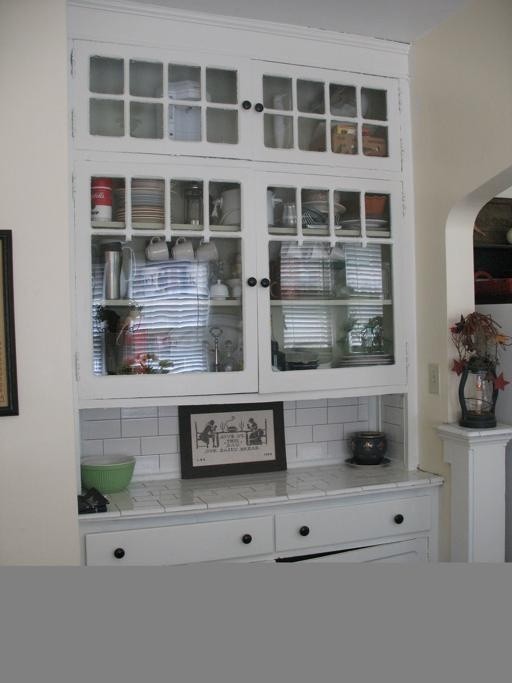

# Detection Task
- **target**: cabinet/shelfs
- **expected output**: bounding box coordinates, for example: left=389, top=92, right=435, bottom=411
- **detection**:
left=69, top=148, right=423, bottom=399
left=68, top=2, right=414, bottom=171
left=67, top=405, right=444, bottom=566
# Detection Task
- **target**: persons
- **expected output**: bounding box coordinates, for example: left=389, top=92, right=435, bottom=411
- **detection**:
left=246, top=417, right=262, bottom=445
left=199, top=420, right=217, bottom=448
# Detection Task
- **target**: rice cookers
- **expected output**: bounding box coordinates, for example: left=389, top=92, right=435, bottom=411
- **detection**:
left=212, top=184, right=282, bottom=227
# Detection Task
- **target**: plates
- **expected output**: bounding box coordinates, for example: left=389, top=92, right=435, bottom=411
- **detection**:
left=345, top=458, right=391, bottom=468
left=341, top=219, right=390, bottom=230
left=114, top=178, right=176, bottom=224
left=302, top=200, right=347, bottom=216
left=338, top=353, right=394, bottom=368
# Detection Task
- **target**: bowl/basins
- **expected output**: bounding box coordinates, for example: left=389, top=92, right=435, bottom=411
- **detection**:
left=80, top=453, right=135, bottom=494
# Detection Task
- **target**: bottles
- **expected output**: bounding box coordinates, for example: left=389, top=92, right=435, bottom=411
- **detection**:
left=184, top=185, right=204, bottom=226
left=97, top=310, right=149, bottom=375
left=212, top=336, right=238, bottom=372
left=91, top=179, right=112, bottom=221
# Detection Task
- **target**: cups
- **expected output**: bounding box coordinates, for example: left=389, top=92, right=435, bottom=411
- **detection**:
left=101, top=242, right=123, bottom=300
left=352, top=432, right=387, bottom=464
left=144, top=235, right=220, bottom=261
